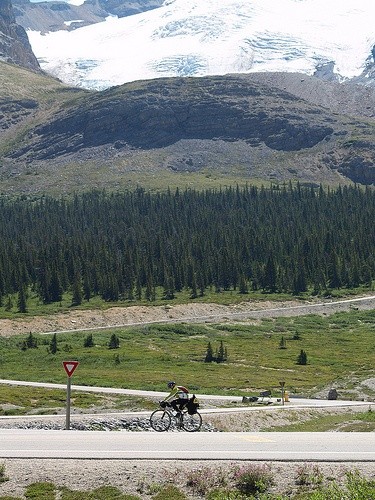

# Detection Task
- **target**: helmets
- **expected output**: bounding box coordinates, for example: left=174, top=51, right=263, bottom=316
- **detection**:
left=168, top=382, right=175, bottom=389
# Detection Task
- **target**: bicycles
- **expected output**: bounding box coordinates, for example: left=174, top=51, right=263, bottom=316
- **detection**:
left=150, top=401, right=202, bottom=432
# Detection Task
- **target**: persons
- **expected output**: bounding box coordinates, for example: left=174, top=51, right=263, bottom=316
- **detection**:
left=160, top=381, right=190, bottom=418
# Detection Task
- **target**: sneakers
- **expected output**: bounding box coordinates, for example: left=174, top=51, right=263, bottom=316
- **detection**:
left=175, top=410, right=183, bottom=418
